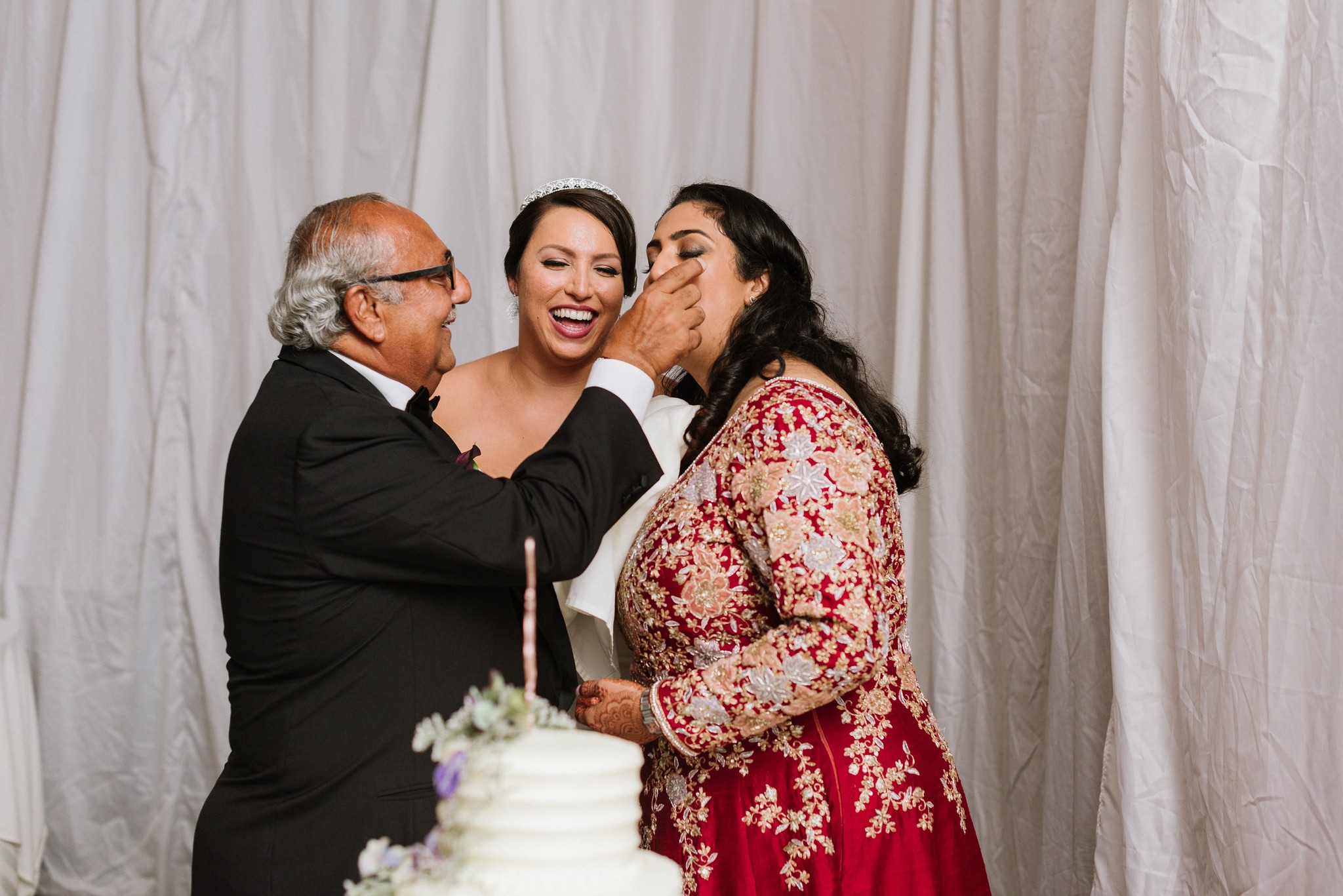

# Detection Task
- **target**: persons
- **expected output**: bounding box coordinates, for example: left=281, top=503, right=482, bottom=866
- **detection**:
left=191, top=189, right=708, bottom=896
left=572, top=180, right=994, bottom=896
left=426, top=173, right=704, bottom=724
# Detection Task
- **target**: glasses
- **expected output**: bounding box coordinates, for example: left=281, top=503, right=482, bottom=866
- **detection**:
left=337, top=256, right=457, bottom=290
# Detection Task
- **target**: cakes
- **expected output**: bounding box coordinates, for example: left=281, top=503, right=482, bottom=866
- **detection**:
left=357, top=727, right=646, bottom=894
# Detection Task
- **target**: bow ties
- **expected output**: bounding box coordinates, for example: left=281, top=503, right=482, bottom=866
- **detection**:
left=405, top=385, right=441, bottom=427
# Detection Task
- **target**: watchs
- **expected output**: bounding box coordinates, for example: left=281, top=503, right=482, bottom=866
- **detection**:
left=640, top=688, right=665, bottom=737
